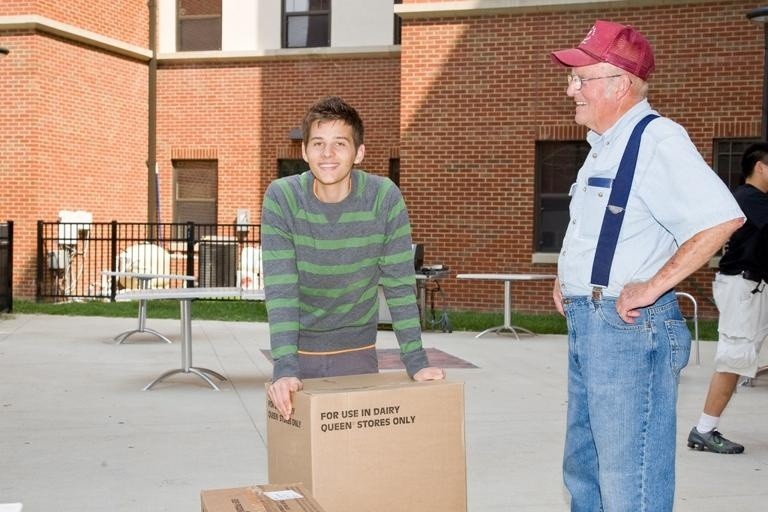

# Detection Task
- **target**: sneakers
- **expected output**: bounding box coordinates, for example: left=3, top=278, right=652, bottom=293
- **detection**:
left=687, top=426, right=744, bottom=454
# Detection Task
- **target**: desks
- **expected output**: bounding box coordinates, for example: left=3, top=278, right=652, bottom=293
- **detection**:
left=374, top=266, right=449, bottom=331
left=96, top=271, right=267, bottom=393
left=457, top=271, right=557, bottom=341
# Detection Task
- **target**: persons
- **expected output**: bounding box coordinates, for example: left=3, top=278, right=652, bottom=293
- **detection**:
left=687, top=142, right=768, bottom=455
left=550, top=21, right=748, bottom=512
left=259, top=98, right=444, bottom=422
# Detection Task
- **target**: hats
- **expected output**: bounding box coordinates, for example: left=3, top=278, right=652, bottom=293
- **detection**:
left=551, top=20, right=655, bottom=81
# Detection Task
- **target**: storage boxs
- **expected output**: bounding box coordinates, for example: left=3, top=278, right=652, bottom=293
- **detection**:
left=200, top=371, right=468, bottom=512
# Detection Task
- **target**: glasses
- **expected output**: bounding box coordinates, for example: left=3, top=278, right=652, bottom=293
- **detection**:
left=567, top=73, right=632, bottom=89
